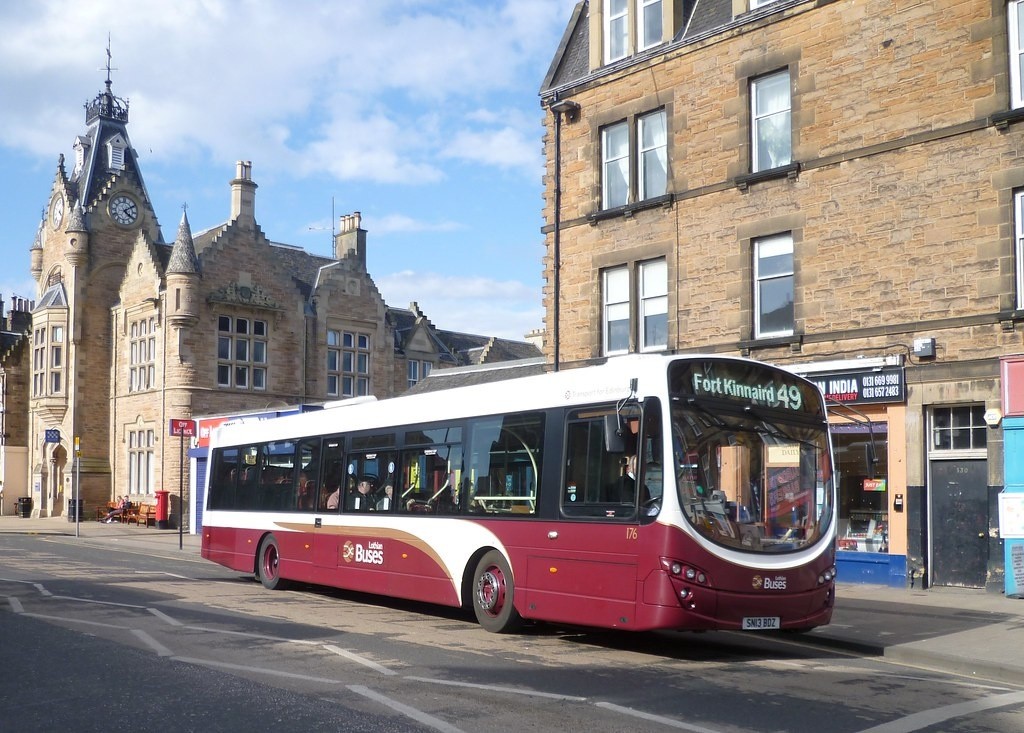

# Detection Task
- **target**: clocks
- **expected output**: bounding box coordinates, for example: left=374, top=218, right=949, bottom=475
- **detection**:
left=111, top=197, right=138, bottom=224
left=52, top=198, right=63, bottom=227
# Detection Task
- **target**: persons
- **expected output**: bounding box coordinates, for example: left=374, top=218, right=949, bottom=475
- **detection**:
left=326, top=477, right=403, bottom=511
left=607, top=455, right=651, bottom=518
left=99, top=495, right=132, bottom=523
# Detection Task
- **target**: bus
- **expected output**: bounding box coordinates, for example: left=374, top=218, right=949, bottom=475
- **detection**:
left=202, top=354, right=879, bottom=635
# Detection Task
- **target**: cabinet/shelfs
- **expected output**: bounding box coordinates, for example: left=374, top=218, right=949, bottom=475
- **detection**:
left=846, top=510, right=889, bottom=552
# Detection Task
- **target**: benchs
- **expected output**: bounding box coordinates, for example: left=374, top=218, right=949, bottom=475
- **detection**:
left=97, top=501, right=155, bottom=528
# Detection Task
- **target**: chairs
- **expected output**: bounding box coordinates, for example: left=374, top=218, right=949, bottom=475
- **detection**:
left=279, top=479, right=431, bottom=511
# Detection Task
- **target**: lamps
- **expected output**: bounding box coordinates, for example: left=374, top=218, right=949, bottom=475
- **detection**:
left=550, top=100, right=581, bottom=119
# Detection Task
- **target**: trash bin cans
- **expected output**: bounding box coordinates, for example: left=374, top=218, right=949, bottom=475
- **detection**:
left=68, top=499, right=84, bottom=523
left=17, top=497, right=31, bottom=518
